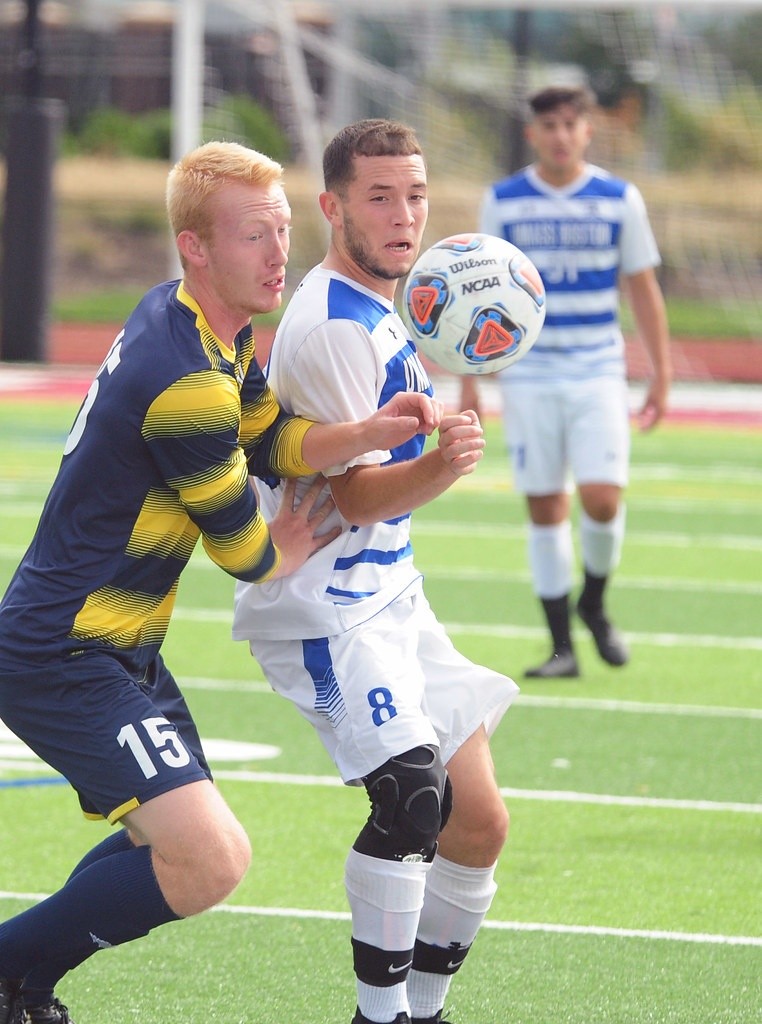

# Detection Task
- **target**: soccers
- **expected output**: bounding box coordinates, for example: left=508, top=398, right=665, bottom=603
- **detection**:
left=400, top=231, right=548, bottom=375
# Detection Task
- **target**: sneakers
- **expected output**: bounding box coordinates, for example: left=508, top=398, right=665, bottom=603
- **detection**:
left=0, top=979, right=29, bottom=1024
left=23, top=988, right=76, bottom=1024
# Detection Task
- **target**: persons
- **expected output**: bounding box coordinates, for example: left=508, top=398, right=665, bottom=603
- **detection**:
left=232, top=120, right=523, bottom=1024
left=459, top=87, right=671, bottom=679
left=0, top=139, right=439, bottom=1024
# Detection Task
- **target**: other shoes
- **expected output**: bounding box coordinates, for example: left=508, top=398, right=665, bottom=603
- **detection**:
left=577, top=594, right=627, bottom=665
left=526, top=647, right=579, bottom=678
